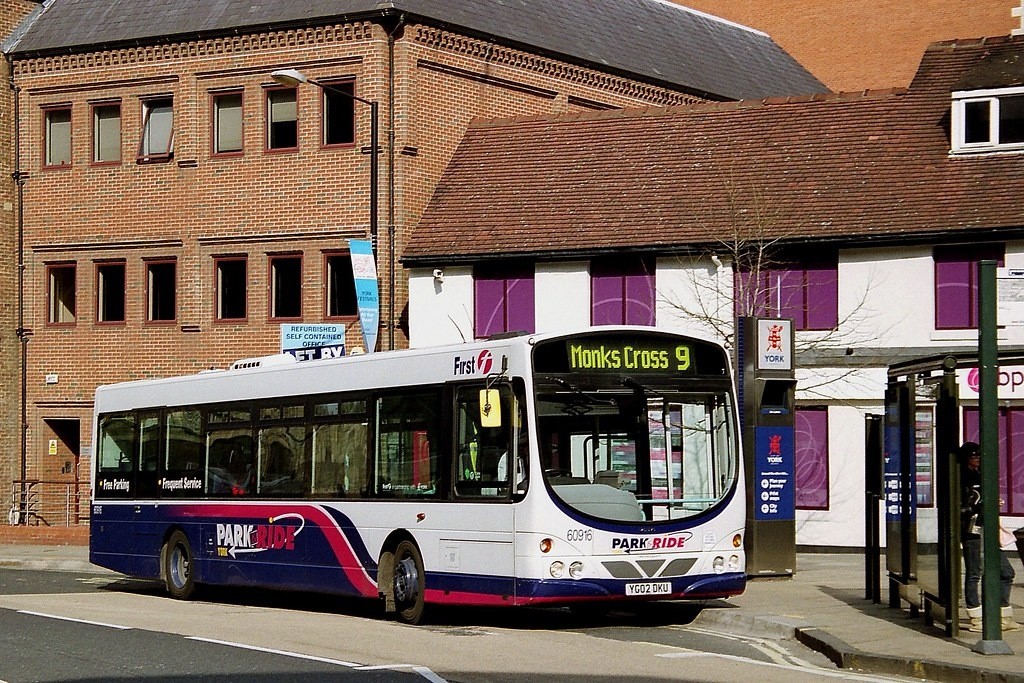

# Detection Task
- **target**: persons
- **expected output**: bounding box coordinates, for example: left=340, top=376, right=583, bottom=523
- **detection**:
left=960, top=441, right=1024, bottom=632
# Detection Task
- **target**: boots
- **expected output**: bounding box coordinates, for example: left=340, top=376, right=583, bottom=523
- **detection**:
left=1001, top=605, right=1023, bottom=633
left=967, top=605, right=983, bottom=633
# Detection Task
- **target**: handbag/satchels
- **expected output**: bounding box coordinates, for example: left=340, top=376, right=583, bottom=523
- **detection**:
left=998, top=519, right=1018, bottom=548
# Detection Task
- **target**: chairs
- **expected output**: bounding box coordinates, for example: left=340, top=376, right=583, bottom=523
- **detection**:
left=594, top=471, right=620, bottom=489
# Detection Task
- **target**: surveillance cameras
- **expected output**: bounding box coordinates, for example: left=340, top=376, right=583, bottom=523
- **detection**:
left=433, top=270, right=444, bottom=278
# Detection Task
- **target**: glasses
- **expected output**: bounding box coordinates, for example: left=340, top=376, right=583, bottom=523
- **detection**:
left=970, top=453, right=982, bottom=460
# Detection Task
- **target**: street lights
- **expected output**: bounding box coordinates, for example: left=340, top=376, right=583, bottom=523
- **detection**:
left=270, top=68, right=378, bottom=271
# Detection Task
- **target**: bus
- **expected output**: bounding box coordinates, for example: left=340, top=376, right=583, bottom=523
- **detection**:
left=88, top=325, right=748, bottom=626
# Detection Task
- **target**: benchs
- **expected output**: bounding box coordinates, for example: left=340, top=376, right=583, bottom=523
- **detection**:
left=892, top=573, right=945, bottom=624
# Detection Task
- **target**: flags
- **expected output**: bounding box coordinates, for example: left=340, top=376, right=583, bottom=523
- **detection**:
left=349, top=238, right=379, bottom=353
